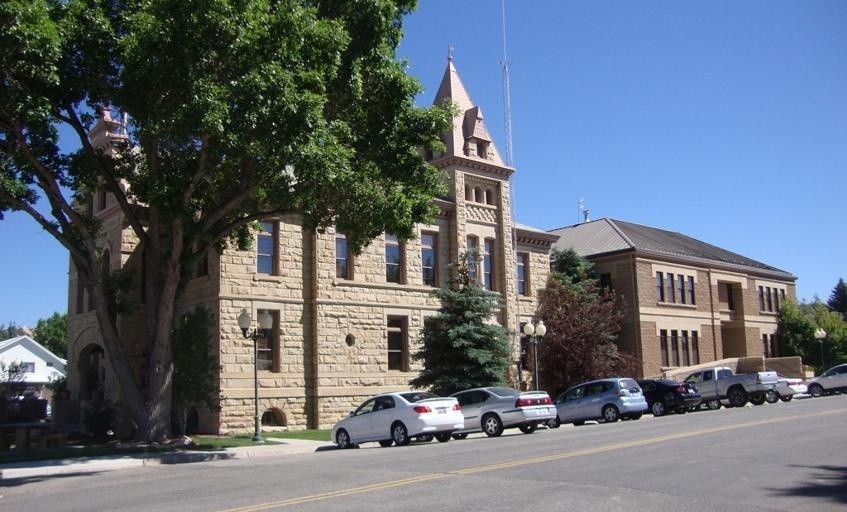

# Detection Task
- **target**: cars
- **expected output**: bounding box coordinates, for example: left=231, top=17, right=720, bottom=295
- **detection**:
left=638, top=377, right=701, bottom=418
left=805, top=363, right=847, bottom=399
left=327, top=389, right=465, bottom=449
left=416, top=384, right=558, bottom=441
left=707, top=375, right=808, bottom=410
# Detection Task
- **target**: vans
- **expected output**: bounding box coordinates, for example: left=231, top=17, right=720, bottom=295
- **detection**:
left=546, top=378, right=649, bottom=429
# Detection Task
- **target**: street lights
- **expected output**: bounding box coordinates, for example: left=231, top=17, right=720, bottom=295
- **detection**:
left=522, top=318, right=548, bottom=392
left=813, top=326, right=827, bottom=374
left=233, top=309, right=278, bottom=445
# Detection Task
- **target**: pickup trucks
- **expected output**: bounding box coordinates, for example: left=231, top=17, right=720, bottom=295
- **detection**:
left=683, top=367, right=780, bottom=411
